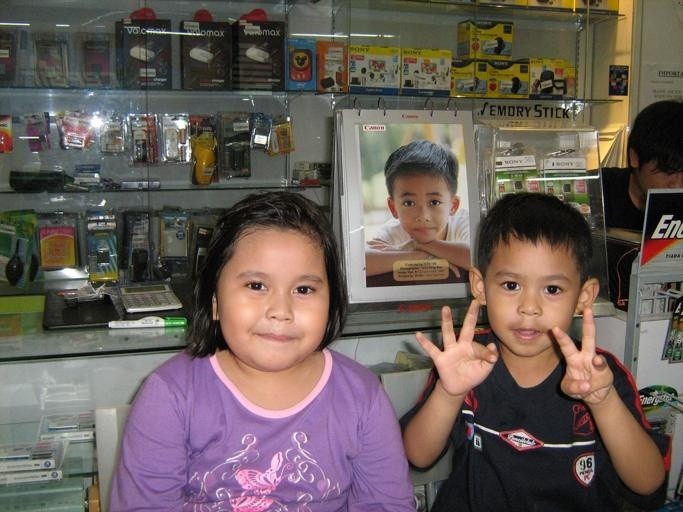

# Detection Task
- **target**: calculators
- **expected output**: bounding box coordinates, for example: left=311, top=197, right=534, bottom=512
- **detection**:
left=117, top=280, right=184, bottom=313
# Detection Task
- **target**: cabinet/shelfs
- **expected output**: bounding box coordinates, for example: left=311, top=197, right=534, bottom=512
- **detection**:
left=1, top=0, right=627, bottom=360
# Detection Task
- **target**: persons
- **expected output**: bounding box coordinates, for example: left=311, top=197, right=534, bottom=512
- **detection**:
left=398, top=194, right=671, bottom=512
left=108, top=193, right=415, bottom=512
left=585, top=101, right=683, bottom=310
left=365, top=139, right=471, bottom=279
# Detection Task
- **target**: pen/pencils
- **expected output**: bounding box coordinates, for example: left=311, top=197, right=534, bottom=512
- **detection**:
left=108, top=316, right=187, bottom=329
